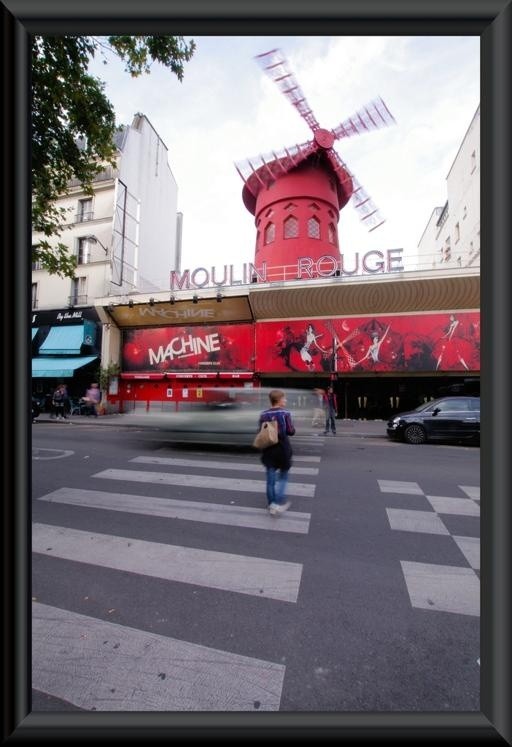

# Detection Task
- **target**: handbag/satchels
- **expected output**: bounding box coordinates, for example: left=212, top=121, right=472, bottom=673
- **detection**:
left=253, top=420, right=278, bottom=449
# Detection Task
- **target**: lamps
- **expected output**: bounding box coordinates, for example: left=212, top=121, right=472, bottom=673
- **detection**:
left=108, top=293, right=226, bottom=312
left=85, top=234, right=108, bottom=256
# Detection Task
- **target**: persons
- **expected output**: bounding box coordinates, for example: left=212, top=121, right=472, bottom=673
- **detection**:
left=258, top=388, right=295, bottom=517
left=320, top=385, right=339, bottom=436
left=32, top=398, right=41, bottom=424
left=353, top=320, right=393, bottom=371
left=300, top=323, right=328, bottom=372
left=430, top=313, right=479, bottom=371
left=85, top=383, right=101, bottom=418
left=51, top=385, right=68, bottom=419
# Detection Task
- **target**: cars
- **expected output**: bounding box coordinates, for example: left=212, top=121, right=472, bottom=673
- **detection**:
left=387, top=396, right=480, bottom=445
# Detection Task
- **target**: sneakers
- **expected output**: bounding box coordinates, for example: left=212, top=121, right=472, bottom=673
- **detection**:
left=269, top=502, right=290, bottom=516
